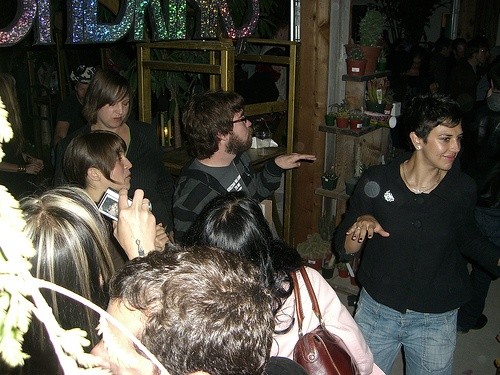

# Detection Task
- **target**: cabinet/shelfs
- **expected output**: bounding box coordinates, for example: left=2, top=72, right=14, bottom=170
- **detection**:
left=137, top=38, right=300, bottom=247
left=315, top=71, right=393, bottom=297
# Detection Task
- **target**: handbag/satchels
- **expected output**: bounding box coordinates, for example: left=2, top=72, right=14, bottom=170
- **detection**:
left=290, top=264, right=361, bottom=375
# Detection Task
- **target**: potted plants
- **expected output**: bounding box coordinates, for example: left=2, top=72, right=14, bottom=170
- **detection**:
left=325, top=78, right=401, bottom=131
left=344, top=7, right=391, bottom=75
left=297, top=208, right=357, bottom=284
left=321, top=168, right=358, bottom=195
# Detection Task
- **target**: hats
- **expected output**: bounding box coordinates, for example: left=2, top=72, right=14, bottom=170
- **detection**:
left=70, top=65, right=96, bottom=84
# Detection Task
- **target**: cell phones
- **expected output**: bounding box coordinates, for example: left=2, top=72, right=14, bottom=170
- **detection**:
left=98, top=187, right=133, bottom=221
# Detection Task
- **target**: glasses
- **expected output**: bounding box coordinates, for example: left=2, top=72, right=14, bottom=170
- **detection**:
left=232, top=114, right=246, bottom=125
left=481, top=49, right=487, bottom=53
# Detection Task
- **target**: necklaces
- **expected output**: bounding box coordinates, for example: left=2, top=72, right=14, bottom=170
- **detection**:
left=402, top=159, right=441, bottom=193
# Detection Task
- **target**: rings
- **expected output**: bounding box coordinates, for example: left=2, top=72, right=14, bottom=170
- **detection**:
left=356, top=226, right=361, bottom=230
left=140, top=202, right=152, bottom=212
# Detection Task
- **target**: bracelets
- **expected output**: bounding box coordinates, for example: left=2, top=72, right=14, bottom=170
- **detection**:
left=132, top=239, right=153, bottom=259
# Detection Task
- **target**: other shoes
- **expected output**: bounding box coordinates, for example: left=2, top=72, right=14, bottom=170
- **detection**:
left=457, top=313, right=488, bottom=334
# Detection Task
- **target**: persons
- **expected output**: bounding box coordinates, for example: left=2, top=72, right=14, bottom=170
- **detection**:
left=0, top=59, right=383, bottom=375
left=331, top=107, right=469, bottom=375
left=375, top=37, right=500, bottom=333
